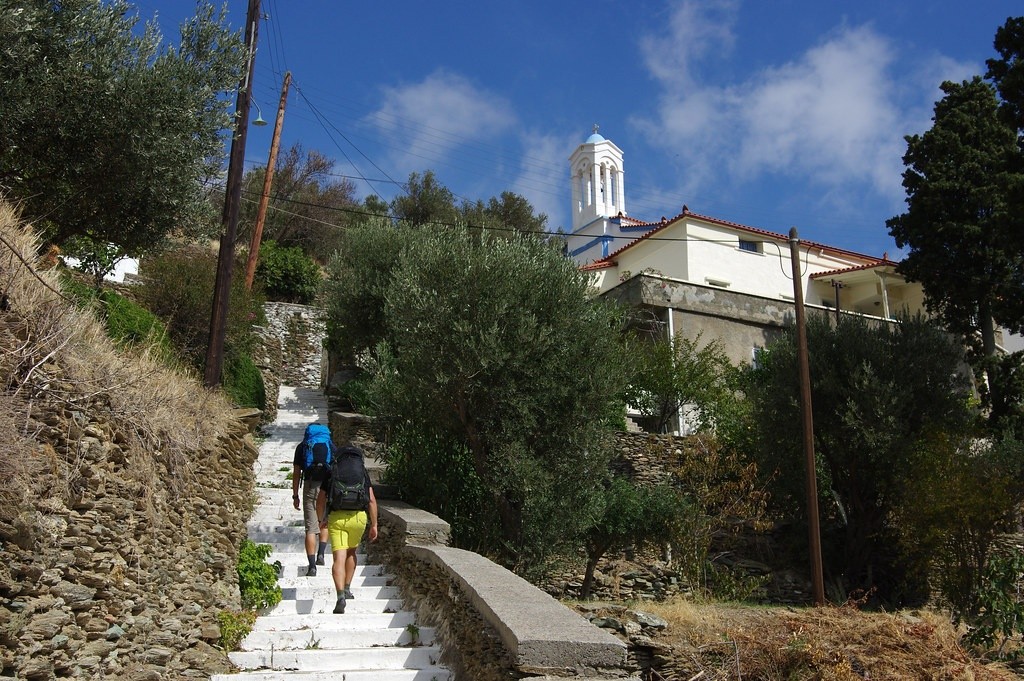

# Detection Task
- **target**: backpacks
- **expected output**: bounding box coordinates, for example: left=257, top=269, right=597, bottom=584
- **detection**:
left=329, top=445, right=370, bottom=510
left=301, top=424, right=334, bottom=469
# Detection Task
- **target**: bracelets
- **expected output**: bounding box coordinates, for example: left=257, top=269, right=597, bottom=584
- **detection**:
left=318, top=520, right=321, bottom=522
left=293, top=495, right=298, bottom=499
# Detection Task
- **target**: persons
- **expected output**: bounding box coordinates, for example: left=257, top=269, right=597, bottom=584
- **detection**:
left=292, top=423, right=337, bottom=575
left=316, top=473, right=377, bottom=614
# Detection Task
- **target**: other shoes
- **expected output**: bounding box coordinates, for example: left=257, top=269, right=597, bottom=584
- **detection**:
left=305, top=568, right=316, bottom=576
left=315, top=554, right=324, bottom=565
left=344, top=590, right=355, bottom=599
left=333, top=598, right=347, bottom=613
